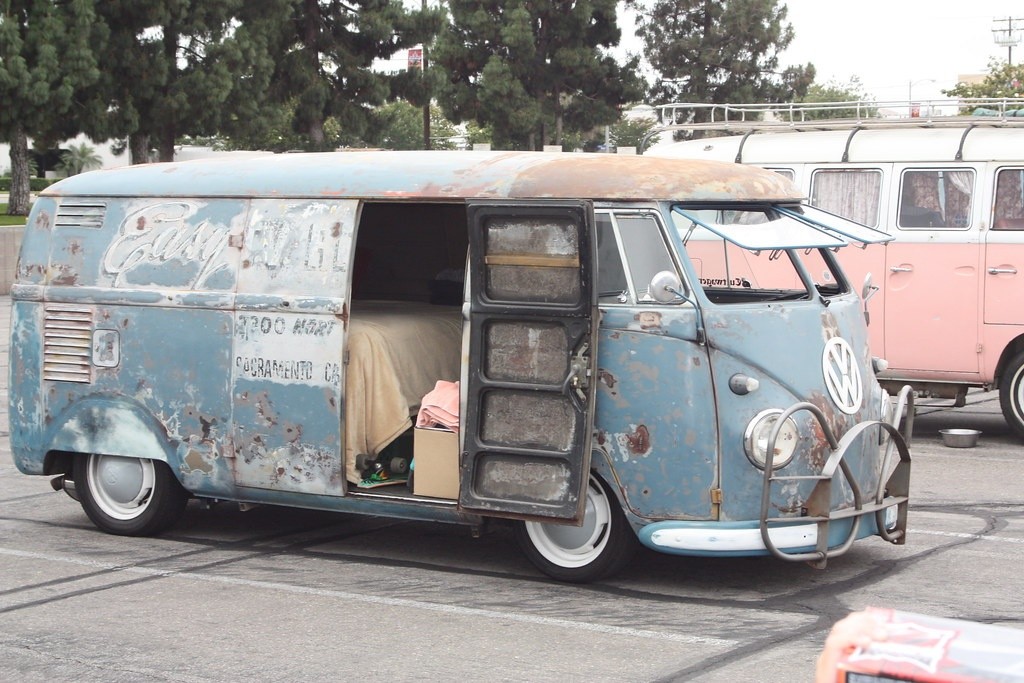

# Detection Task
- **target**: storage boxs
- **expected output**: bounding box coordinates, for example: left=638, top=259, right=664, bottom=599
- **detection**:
left=415, top=427, right=462, bottom=500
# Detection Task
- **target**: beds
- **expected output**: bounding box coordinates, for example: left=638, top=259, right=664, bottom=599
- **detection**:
left=350, top=299, right=463, bottom=481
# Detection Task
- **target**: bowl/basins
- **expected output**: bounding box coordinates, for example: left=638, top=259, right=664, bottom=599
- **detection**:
left=939, top=430, right=983, bottom=447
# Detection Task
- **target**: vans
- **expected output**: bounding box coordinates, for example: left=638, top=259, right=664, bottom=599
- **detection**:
left=635, top=96, right=1024, bottom=447
left=9, top=152, right=914, bottom=586
